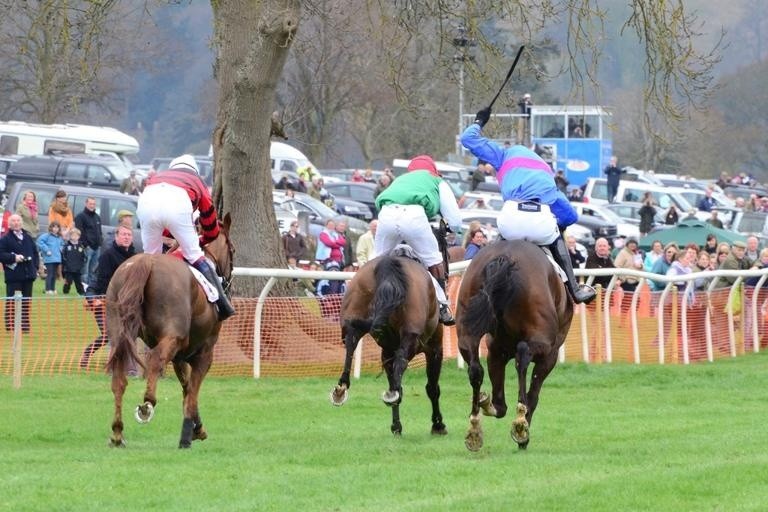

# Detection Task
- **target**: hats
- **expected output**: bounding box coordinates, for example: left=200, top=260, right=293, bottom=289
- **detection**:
left=732, top=241, right=746, bottom=248
left=117, top=209, right=134, bottom=218
left=625, top=236, right=637, bottom=244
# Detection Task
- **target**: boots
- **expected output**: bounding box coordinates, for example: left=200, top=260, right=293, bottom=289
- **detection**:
left=192, top=255, right=236, bottom=321
left=428, top=262, right=459, bottom=326
left=549, top=234, right=596, bottom=305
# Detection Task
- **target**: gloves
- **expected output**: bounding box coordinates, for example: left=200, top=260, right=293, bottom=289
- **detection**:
left=474, top=107, right=491, bottom=128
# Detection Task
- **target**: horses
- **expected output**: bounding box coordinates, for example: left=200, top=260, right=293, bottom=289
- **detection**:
left=455, top=238, right=574, bottom=453
left=329, top=219, right=449, bottom=438
left=102, top=211, right=235, bottom=451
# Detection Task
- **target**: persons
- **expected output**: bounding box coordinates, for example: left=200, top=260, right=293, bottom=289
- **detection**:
left=636, top=168, right=768, bottom=234
left=445, top=158, right=497, bottom=262
left=0, top=187, right=135, bottom=369
left=275, top=166, right=395, bottom=320
left=369, top=154, right=461, bottom=326
left=136, top=154, right=235, bottom=319
left=517, top=92, right=629, bottom=206
left=565, top=236, right=768, bottom=361
left=461, top=107, right=595, bottom=304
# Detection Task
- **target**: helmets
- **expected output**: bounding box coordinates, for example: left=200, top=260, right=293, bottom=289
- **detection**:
left=407, top=155, right=437, bottom=173
left=168, top=154, right=198, bottom=175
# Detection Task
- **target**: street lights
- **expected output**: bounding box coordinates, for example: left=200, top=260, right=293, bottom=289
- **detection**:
left=450, top=24, right=478, bottom=157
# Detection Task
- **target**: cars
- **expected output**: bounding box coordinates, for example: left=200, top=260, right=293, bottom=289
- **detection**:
left=0, top=121, right=768, bottom=282
